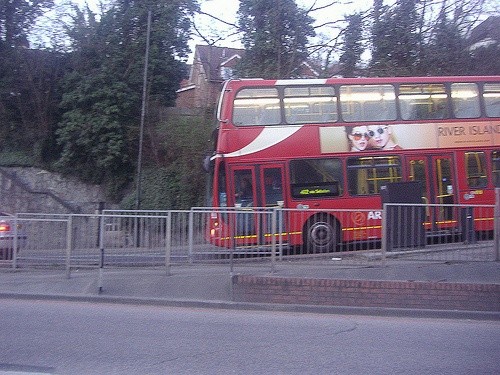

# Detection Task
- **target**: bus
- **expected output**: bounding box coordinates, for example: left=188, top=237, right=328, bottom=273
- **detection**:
left=203, top=73, right=499, bottom=252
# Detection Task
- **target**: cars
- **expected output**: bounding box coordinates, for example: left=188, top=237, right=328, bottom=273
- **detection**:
left=0, top=212, right=24, bottom=260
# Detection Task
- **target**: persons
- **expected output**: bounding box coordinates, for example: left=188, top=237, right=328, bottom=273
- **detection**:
left=344, top=125, right=369, bottom=152
left=364, top=125, right=403, bottom=151
left=232, top=171, right=253, bottom=200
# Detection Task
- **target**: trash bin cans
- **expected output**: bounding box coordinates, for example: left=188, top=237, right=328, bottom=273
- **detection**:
left=460, top=206, right=474, bottom=243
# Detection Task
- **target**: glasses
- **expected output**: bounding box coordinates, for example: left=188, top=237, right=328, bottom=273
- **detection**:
left=368, top=126, right=388, bottom=136
left=350, top=132, right=370, bottom=141
